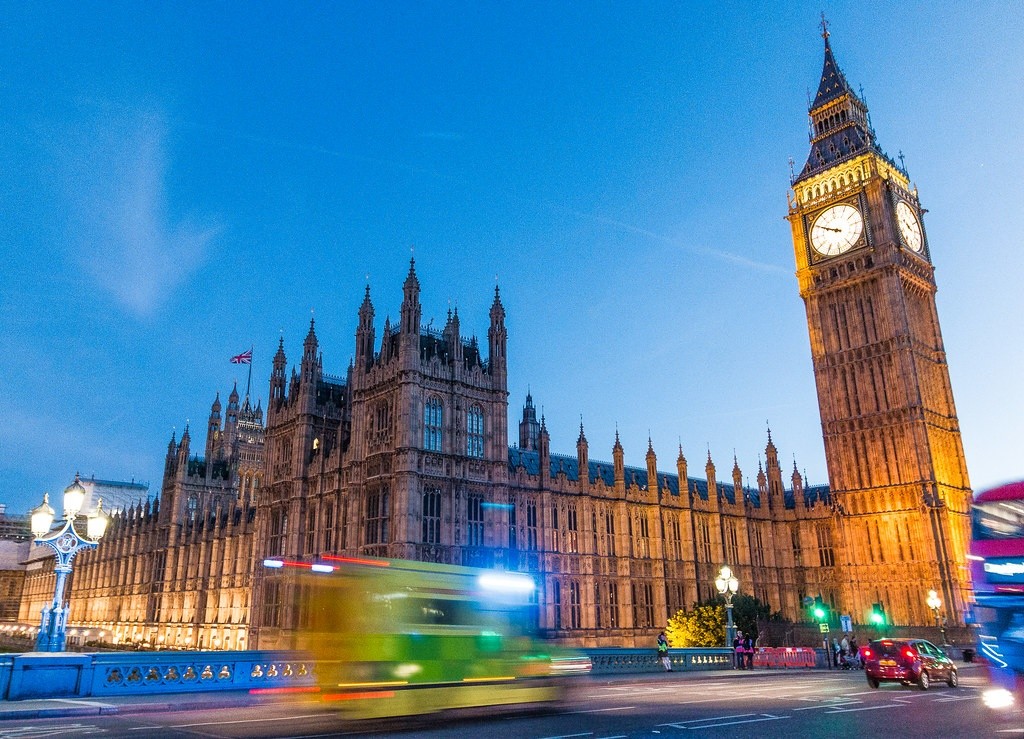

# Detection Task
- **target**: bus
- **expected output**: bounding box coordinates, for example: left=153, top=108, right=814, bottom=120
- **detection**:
left=967, top=480, right=1023, bottom=702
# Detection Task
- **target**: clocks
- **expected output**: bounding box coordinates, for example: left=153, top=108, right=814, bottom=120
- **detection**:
left=894, top=199, right=925, bottom=254
left=807, top=202, right=865, bottom=257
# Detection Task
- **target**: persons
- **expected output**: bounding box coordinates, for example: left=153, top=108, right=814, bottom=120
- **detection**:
left=833, top=634, right=872, bottom=670
left=655, top=632, right=673, bottom=672
left=733, top=633, right=756, bottom=670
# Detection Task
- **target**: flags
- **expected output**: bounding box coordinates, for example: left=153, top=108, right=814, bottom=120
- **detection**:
left=230, top=349, right=252, bottom=364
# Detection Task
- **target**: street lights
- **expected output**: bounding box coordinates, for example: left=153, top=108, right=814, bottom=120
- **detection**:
left=815, top=608, right=832, bottom=670
left=715, top=562, right=739, bottom=647
left=927, top=590, right=941, bottom=627
left=31, top=471, right=109, bottom=652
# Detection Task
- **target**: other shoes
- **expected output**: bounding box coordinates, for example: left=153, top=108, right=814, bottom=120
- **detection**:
left=743, top=668, right=747, bottom=670
left=748, top=668, right=750, bottom=671
left=738, top=667, right=741, bottom=670
left=667, top=669, right=674, bottom=672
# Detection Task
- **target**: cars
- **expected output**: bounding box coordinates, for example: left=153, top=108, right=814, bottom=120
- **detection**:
left=864, top=638, right=957, bottom=690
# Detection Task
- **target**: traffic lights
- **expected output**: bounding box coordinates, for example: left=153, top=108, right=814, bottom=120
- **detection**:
left=871, top=604, right=886, bottom=627
